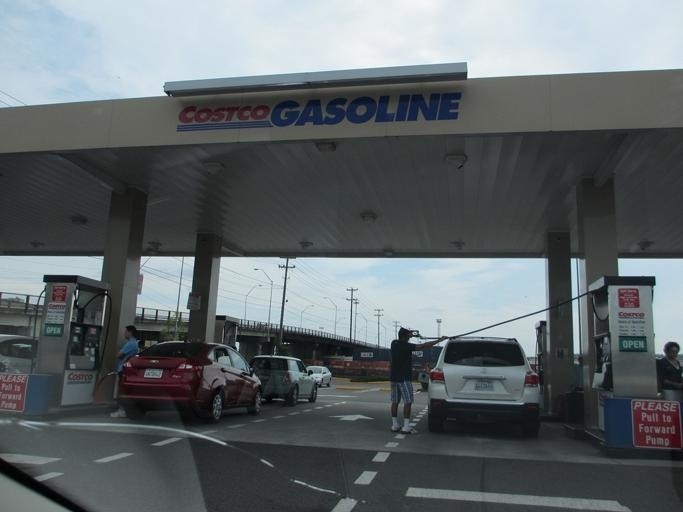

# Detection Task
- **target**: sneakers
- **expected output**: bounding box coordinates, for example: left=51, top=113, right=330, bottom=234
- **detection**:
left=109, top=407, right=127, bottom=418
left=390, top=425, right=400, bottom=432
left=400, top=426, right=417, bottom=435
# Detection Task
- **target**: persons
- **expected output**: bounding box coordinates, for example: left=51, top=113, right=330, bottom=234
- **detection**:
left=110, top=325, right=138, bottom=417
left=389, top=327, right=448, bottom=434
left=657, top=342, right=683, bottom=403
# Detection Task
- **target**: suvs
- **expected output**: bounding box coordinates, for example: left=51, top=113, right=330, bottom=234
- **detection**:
left=427, top=336, right=540, bottom=440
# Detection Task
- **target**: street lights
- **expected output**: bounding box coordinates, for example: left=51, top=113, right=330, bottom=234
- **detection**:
left=300, top=304, right=314, bottom=330
left=323, top=296, right=337, bottom=339
left=244, top=284, right=262, bottom=325
left=254, top=268, right=274, bottom=336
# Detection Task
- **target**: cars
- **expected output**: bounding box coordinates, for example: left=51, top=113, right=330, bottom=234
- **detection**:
left=0, top=334, right=38, bottom=375
left=307, top=365, right=332, bottom=388
left=116, top=341, right=262, bottom=423
left=250, top=355, right=318, bottom=406
